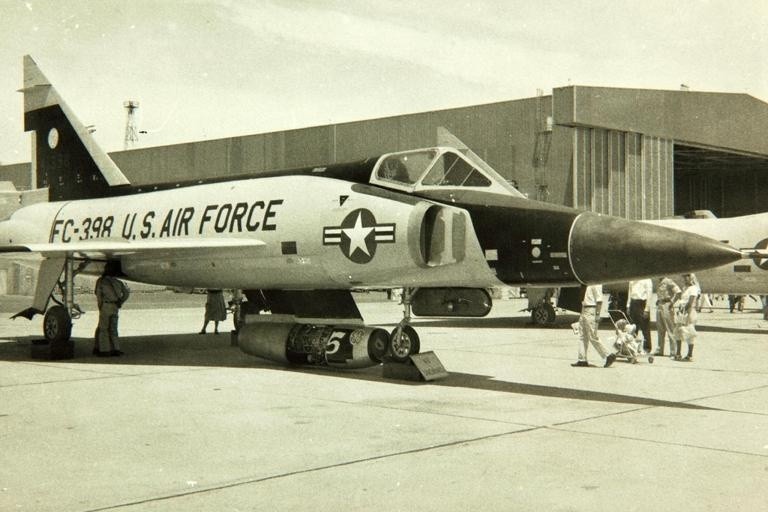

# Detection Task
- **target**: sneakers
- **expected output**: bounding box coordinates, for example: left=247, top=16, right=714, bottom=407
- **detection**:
left=99, top=350, right=123, bottom=358
left=604, top=353, right=616, bottom=367
left=572, top=361, right=588, bottom=367
left=199, top=331, right=219, bottom=334
left=676, top=355, right=692, bottom=362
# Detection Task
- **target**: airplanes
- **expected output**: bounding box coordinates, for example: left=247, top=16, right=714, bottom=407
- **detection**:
left=0, top=55, right=751, bottom=374
left=434, top=127, right=768, bottom=329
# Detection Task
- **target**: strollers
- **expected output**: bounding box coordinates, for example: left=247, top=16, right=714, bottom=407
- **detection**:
left=606, top=309, right=654, bottom=364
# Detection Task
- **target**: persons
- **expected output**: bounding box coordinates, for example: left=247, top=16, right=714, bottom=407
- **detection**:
left=696, top=293, right=714, bottom=313
left=198, top=289, right=227, bottom=334
left=93, top=262, right=130, bottom=357
left=569, top=284, right=617, bottom=369
left=624, top=278, right=654, bottom=356
left=726, top=294, right=736, bottom=313
left=668, top=272, right=703, bottom=362
left=651, top=276, right=683, bottom=360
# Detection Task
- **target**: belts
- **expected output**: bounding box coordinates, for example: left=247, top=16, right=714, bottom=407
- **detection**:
left=105, top=301, right=118, bottom=304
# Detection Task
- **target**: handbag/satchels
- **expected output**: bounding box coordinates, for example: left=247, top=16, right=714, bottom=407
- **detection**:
left=679, top=325, right=696, bottom=340
